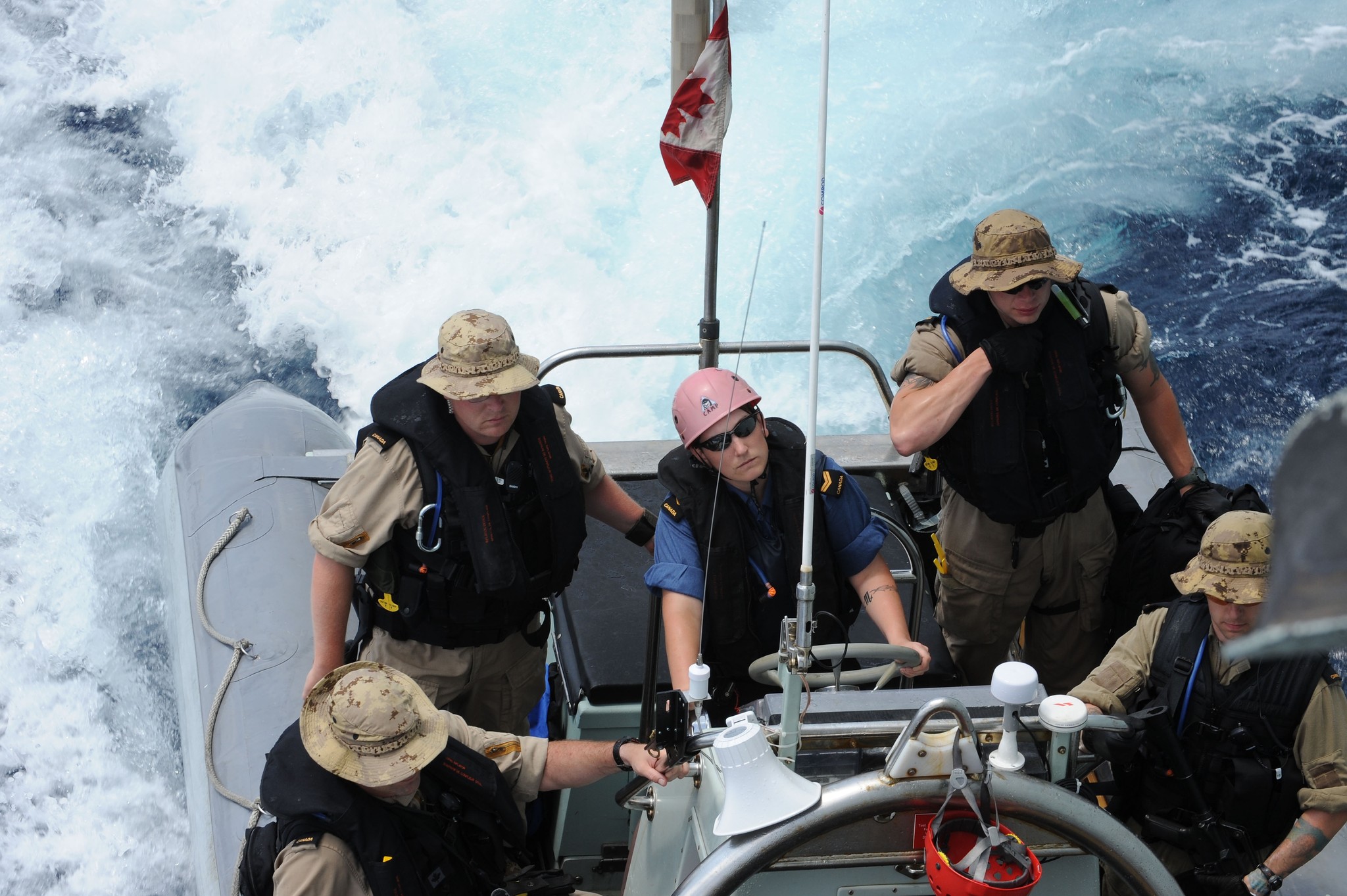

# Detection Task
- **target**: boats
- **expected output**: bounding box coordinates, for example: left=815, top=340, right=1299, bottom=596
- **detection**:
left=153, top=0, right=1347, bottom=896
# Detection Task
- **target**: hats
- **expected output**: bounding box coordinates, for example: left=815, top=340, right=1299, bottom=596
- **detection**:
left=1171, top=510, right=1274, bottom=603
left=949, top=211, right=1081, bottom=295
left=416, top=309, right=541, bottom=400
left=300, top=662, right=448, bottom=786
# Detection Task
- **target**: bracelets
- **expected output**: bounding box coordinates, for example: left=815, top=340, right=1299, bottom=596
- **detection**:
left=625, top=507, right=658, bottom=546
left=613, top=736, right=641, bottom=771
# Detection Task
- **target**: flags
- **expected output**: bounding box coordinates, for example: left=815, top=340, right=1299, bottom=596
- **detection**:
left=659, top=0, right=733, bottom=211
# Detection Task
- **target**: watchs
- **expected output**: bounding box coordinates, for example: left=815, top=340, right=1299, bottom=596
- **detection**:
left=1256, top=862, right=1283, bottom=892
left=1173, top=466, right=1206, bottom=491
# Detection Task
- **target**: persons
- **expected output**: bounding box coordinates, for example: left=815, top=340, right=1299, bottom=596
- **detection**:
left=644, top=367, right=932, bottom=712
left=889, top=208, right=1208, bottom=697
left=260, top=659, right=691, bottom=896
left=1066, top=510, right=1347, bottom=896
left=301, top=310, right=658, bottom=838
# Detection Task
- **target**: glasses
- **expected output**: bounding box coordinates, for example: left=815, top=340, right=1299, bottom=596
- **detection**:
left=1004, top=279, right=1048, bottom=295
left=693, top=413, right=756, bottom=452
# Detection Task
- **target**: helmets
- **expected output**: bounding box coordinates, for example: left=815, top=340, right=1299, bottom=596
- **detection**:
left=672, top=367, right=762, bottom=449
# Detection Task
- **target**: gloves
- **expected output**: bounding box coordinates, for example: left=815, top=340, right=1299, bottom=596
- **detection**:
left=1083, top=714, right=1149, bottom=764
left=979, top=325, right=1045, bottom=374
